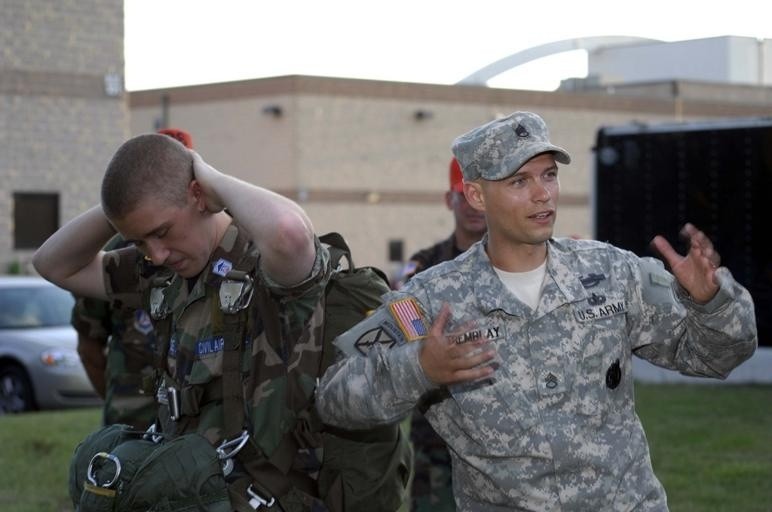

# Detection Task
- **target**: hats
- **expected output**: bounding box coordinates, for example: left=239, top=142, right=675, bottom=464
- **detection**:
left=450, top=111, right=572, bottom=191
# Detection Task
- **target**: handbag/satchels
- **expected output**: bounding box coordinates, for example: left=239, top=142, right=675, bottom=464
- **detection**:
left=65, top=422, right=238, bottom=511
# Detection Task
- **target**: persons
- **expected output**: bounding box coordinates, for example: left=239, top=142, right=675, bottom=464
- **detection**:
left=66, top=127, right=192, bottom=404
left=312, top=112, right=760, bottom=512
left=396, top=155, right=486, bottom=510
left=30, top=130, right=327, bottom=512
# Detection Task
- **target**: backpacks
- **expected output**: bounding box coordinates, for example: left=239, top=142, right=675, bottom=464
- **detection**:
left=221, top=230, right=410, bottom=511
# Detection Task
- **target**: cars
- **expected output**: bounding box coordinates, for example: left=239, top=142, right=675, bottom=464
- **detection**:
left=0, top=273, right=96, bottom=412
